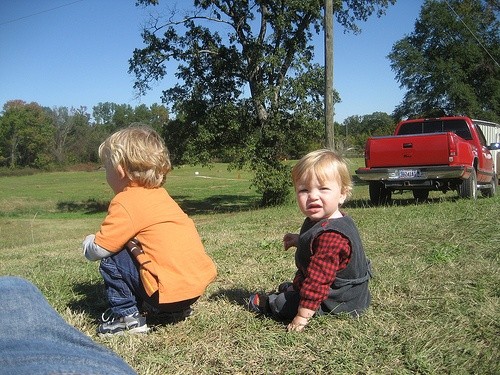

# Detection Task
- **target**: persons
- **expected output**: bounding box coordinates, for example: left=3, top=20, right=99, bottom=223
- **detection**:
left=249, top=148, right=371, bottom=336
left=81, top=121, right=218, bottom=334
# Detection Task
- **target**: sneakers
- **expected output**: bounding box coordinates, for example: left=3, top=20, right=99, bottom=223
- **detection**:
left=96, top=308, right=147, bottom=338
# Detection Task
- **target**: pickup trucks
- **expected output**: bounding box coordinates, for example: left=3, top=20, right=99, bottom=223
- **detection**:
left=351, top=116, right=497, bottom=206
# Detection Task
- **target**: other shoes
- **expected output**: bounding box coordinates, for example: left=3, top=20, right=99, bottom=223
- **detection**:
left=279, top=281, right=296, bottom=293
left=249, top=293, right=268, bottom=313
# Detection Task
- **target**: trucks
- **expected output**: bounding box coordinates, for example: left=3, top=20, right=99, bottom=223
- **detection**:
left=470, top=119, right=500, bottom=188
